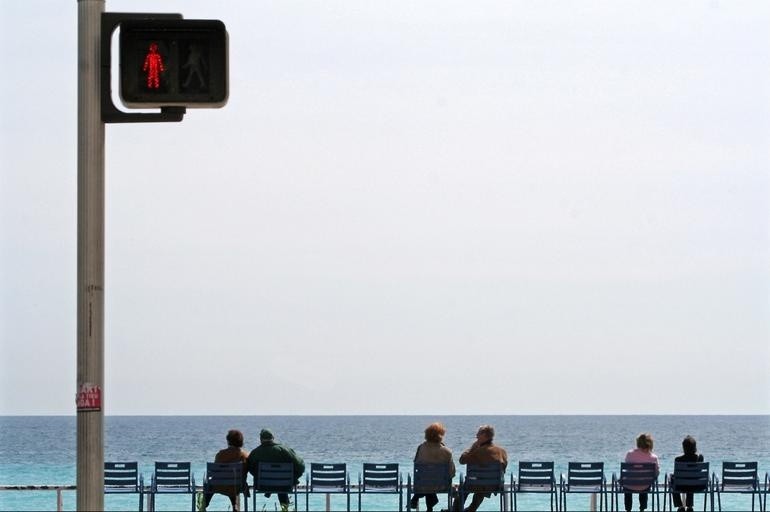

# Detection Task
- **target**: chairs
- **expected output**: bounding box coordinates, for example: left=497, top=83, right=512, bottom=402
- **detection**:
left=201, top=459, right=247, bottom=512
left=663, top=459, right=714, bottom=511
left=610, top=460, right=661, bottom=512
left=304, top=459, right=353, bottom=511
left=713, top=461, right=763, bottom=512
left=763, top=469, right=770, bottom=511
left=459, top=464, right=505, bottom=512
left=510, top=460, right=558, bottom=512
left=148, top=459, right=198, bottom=512
left=407, top=462, right=452, bottom=511
left=357, top=461, right=405, bottom=511
left=99, top=459, right=145, bottom=510
left=252, top=460, right=300, bottom=512
left=559, top=458, right=609, bottom=512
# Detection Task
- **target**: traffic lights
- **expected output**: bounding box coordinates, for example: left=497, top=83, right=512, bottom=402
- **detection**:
left=115, top=17, right=229, bottom=111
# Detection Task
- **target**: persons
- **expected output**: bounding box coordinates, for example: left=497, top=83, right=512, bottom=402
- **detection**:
left=247, top=429, right=305, bottom=511
left=672, top=436, right=704, bottom=512
left=411, top=422, right=456, bottom=512
left=441, top=425, right=508, bottom=512
left=202, top=430, right=249, bottom=512
left=623, top=433, right=660, bottom=512
